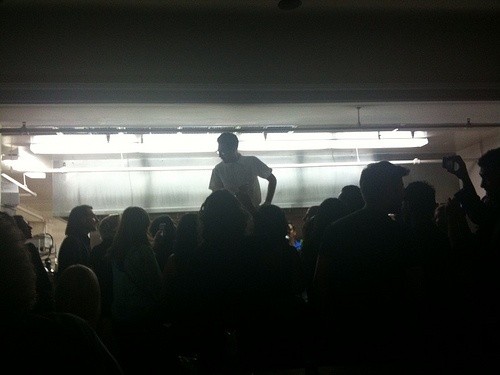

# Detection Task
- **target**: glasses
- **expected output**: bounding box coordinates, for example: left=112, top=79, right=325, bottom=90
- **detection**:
left=216, top=149, right=230, bottom=154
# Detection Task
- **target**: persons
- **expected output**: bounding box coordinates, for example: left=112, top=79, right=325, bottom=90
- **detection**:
left=0, top=147, right=499, bottom=374
left=208, top=132, right=277, bottom=210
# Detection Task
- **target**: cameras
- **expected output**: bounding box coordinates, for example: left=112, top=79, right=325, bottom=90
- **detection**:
left=442, top=157, right=454, bottom=170
